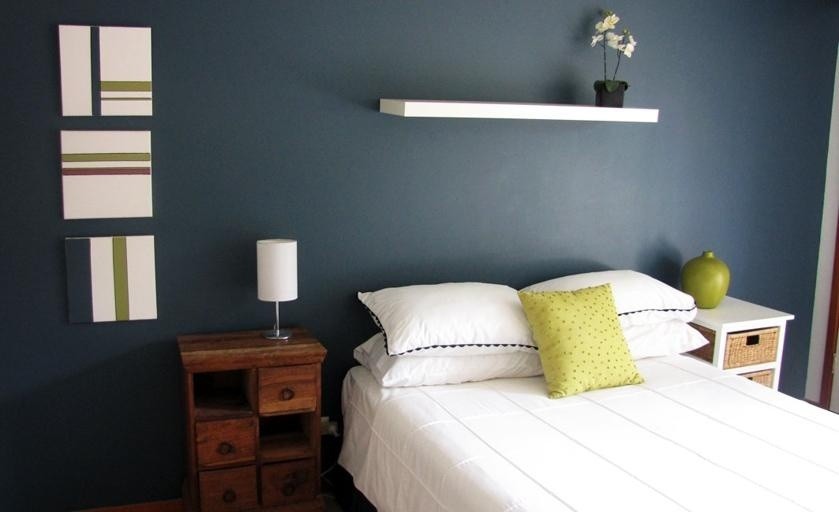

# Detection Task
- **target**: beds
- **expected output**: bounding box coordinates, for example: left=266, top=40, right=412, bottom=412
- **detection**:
left=338, top=357, right=839, bottom=511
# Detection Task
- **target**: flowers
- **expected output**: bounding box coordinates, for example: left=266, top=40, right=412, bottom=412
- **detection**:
left=590, top=9, right=639, bottom=80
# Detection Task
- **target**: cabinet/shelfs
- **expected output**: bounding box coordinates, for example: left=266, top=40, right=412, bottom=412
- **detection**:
left=175, top=326, right=326, bottom=511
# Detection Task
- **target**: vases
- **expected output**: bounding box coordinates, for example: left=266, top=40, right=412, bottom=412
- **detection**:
left=593, top=80, right=629, bottom=108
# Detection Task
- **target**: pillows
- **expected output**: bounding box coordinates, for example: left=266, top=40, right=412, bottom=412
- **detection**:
left=354, top=333, right=545, bottom=387
left=628, top=321, right=711, bottom=360
left=518, top=269, right=699, bottom=335
left=357, top=282, right=538, bottom=352
left=516, top=284, right=646, bottom=400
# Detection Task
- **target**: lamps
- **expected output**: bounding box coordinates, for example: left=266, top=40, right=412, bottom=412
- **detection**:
left=254, top=238, right=299, bottom=340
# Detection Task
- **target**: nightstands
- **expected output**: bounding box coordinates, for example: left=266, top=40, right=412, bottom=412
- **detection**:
left=685, top=295, right=795, bottom=394
